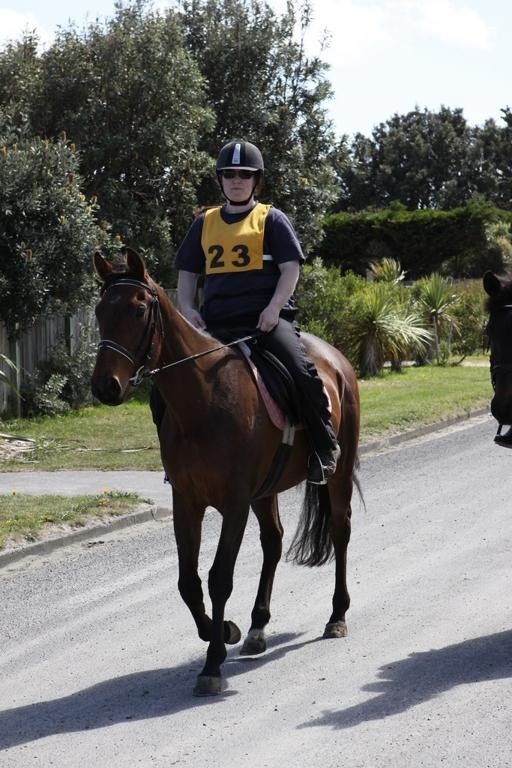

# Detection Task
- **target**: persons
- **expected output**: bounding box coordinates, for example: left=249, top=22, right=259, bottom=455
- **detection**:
left=147, top=140, right=342, bottom=483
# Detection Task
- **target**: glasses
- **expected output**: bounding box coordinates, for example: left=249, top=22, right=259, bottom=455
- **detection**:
left=221, top=169, right=257, bottom=179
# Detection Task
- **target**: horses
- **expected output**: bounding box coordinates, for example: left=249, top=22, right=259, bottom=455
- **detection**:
left=87, top=248, right=363, bottom=696
left=481, top=267, right=512, bottom=428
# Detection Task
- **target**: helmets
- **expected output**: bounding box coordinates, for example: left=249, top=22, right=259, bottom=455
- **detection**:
left=216, top=140, right=264, bottom=176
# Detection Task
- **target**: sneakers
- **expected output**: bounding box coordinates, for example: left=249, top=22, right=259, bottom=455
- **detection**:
left=307, top=444, right=342, bottom=482
